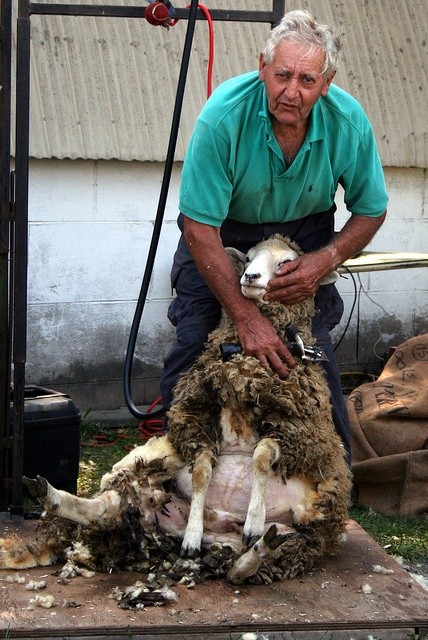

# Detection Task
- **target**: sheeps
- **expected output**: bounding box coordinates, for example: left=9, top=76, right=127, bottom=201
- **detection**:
left=19, top=232, right=354, bottom=586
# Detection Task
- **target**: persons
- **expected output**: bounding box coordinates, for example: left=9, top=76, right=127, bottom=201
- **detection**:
left=152, top=9, right=388, bottom=470
